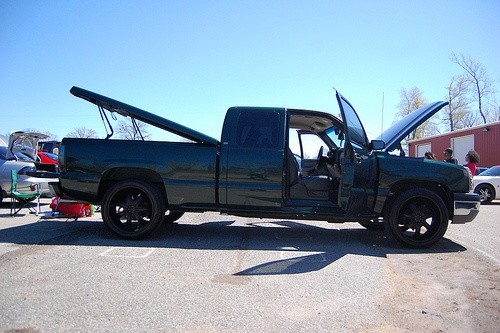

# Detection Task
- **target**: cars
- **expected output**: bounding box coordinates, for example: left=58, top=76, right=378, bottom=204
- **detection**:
left=35, top=151, right=59, bottom=165
left=471, top=165, right=500, bottom=204
left=37, top=140, right=62, bottom=155
left=0, top=130, right=59, bottom=202
left=474, top=166, right=489, bottom=175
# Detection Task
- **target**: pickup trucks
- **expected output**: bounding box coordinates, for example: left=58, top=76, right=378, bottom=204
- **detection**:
left=24, top=85, right=482, bottom=249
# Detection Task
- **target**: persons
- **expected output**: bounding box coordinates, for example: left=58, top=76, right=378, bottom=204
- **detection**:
left=463, top=150, right=480, bottom=176
left=400, top=147, right=405, bottom=157
left=442, top=148, right=458, bottom=164
left=425, top=152, right=436, bottom=159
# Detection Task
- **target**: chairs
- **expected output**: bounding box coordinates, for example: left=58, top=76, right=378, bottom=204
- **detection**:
left=11, top=168, right=40, bottom=216
left=287, top=147, right=330, bottom=200
